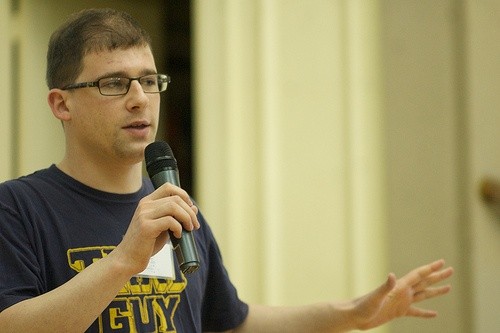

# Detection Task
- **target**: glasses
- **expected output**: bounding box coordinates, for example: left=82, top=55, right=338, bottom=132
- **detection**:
left=61, top=74, right=171, bottom=96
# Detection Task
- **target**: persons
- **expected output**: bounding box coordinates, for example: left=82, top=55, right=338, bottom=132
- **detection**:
left=1, top=8, right=453, bottom=333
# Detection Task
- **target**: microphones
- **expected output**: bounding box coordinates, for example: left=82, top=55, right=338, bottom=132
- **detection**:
left=144, top=141, right=200, bottom=275
